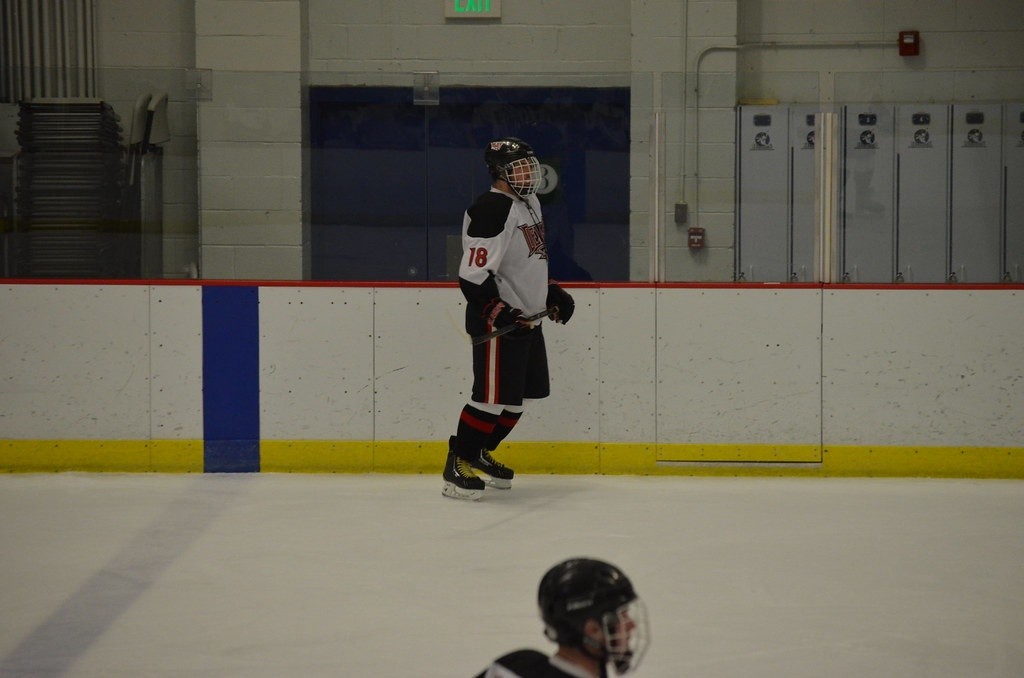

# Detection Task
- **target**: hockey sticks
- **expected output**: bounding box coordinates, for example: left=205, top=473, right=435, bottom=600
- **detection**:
left=474, top=306, right=559, bottom=343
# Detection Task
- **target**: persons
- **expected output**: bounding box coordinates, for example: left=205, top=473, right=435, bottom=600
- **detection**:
left=478, top=558, right=647, bottom=678
left=437, top=136, right=576, bottom=491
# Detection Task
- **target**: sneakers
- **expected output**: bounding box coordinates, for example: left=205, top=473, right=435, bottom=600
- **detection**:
left=442, top=447, right=486, bottom=501
left=472, top=448, right=514, bottom=489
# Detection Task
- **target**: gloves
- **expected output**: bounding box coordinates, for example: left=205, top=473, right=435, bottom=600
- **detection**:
left=546, top=279, right=575, bottom=324
left=482, top=298, right=534, bottom=339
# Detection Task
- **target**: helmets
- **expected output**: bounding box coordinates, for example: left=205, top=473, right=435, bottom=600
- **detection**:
left=485, top=136, right=542, bottom=198
left=538, top=558, right=651, bottom=676
